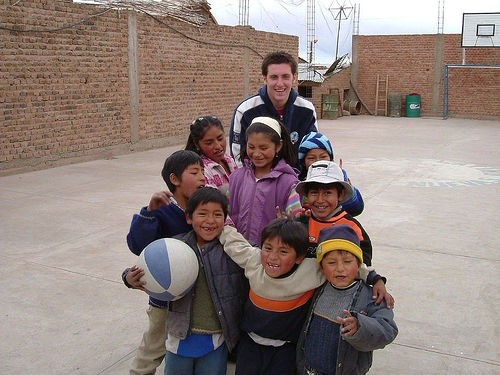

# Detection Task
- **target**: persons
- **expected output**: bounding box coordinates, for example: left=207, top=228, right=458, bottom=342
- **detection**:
left=218, top=217, right=395, bottom=375
left=183, top=115, right=239, bottom=228
left=294, top=224, right=399, bottom=375
left=122, top=187, right=263, bottom=375
left=277, top=160, right=372, bottom=268
left=293, top=131, right=364, bottom=218
left=228, top=51, right=320, bottom=180
left=223, top=116, right=306, bottom=249
left=126, top=150, right=209, bottom=375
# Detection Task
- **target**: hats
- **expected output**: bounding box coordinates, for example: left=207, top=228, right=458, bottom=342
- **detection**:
left=294, top=160, right=354, bottom=205
left=316, top=224, right=363, bottom=273
left=298, top=131, right=334, bottom=162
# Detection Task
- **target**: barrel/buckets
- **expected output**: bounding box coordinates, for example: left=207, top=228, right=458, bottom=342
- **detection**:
left=322, top=94, right=340, bottom=120
left=405, top=95, right=421, bottom=118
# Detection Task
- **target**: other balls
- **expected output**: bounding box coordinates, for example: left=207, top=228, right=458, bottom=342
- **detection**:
left=138, top=236, right=200, bottom=303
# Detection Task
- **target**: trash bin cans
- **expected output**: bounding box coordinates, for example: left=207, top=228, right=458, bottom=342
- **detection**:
left=388, top=92, right=402, bottom=118
left=343, top=99, right=361, bottom=114
left=405, top=94, right=421, bottom=117
left=322, top=93, right=339, bottom=120
